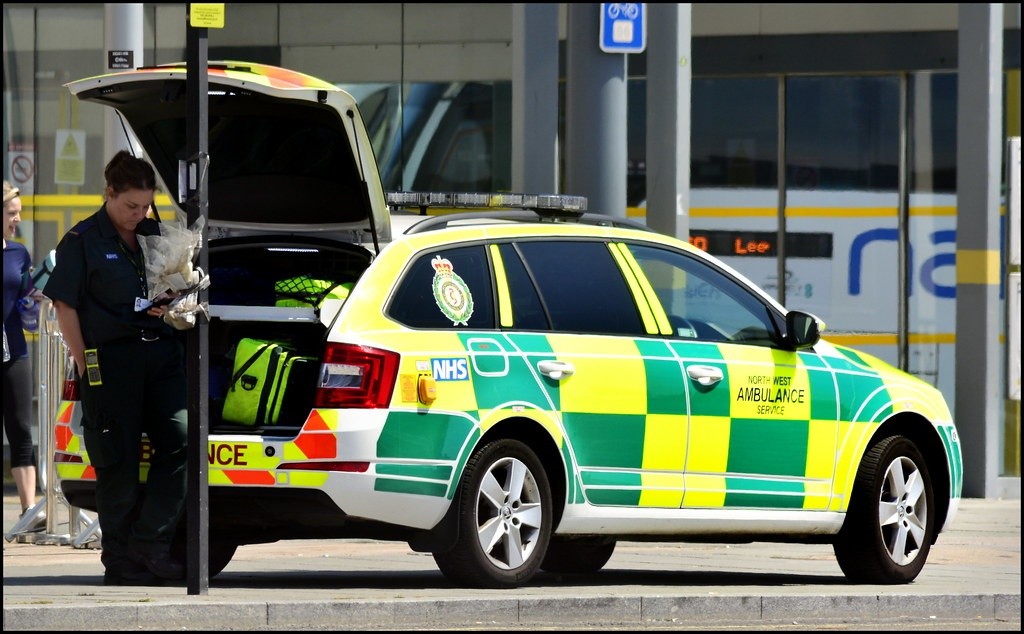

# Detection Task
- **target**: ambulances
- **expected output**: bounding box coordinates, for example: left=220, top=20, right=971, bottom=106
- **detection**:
left=54, top=60, right=963, bottom=589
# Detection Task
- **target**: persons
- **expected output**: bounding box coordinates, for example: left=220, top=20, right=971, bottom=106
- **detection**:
left=41, top=149, right=189, bottom=585
left=3, top=180, right=47, bottom=534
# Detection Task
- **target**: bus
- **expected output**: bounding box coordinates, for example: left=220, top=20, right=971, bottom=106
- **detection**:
left=337, top=30, right=1023, bottom=432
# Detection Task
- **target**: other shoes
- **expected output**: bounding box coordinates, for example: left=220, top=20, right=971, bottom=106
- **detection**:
left=129, top=539, right=185, bottom=580
left=20, top=506, right=47, bottom=531
left=104, top=567, right=172, bottom=586
